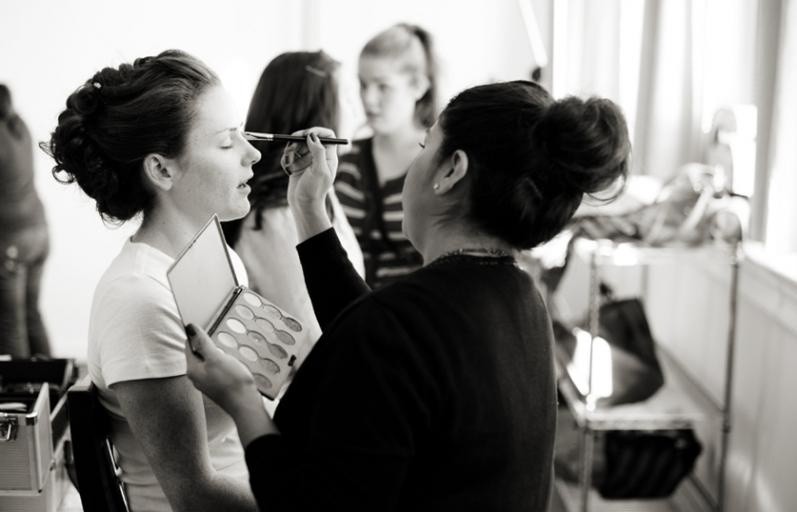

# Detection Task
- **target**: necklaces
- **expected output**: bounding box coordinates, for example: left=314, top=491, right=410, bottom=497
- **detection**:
left=438, top=248, right=509, bottom=257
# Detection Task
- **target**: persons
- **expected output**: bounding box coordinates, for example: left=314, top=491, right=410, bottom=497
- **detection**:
left=328, top=23, right=440, bottom=294
left=185, top=81, right=632, bottom=511
left=1, top=82, right=52, bottom=363
left=217, top=50, right=366, bottom=348
left=37, top=47, right=276, bottom=512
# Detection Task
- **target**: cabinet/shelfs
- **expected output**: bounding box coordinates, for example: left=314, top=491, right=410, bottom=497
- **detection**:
left=553, top=172, right=744, bottom=512
